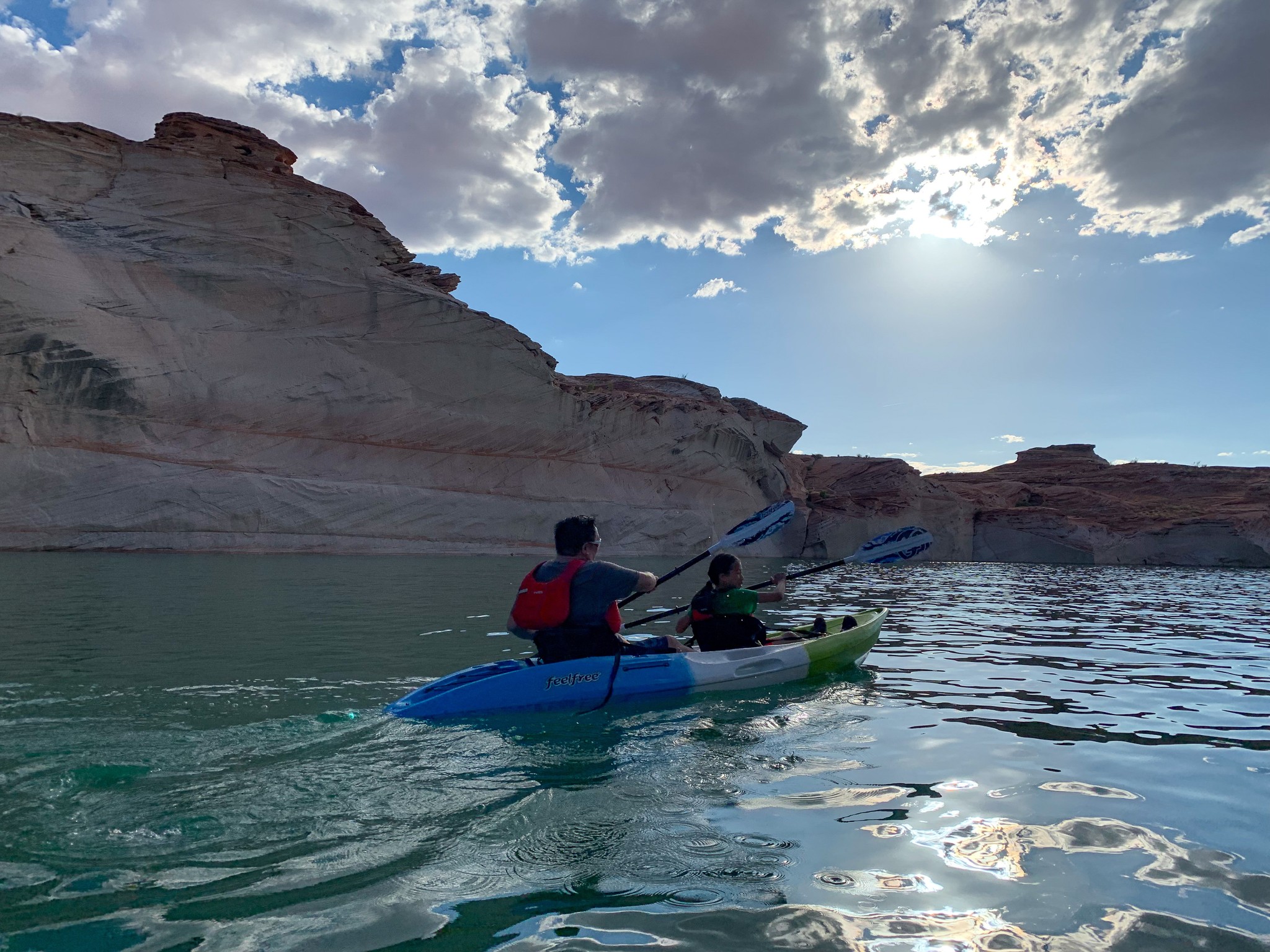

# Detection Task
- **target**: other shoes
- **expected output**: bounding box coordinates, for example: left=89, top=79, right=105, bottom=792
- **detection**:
left=807, top=616, right=827, bottom=639
left=839, top=614, right=858, bottom=632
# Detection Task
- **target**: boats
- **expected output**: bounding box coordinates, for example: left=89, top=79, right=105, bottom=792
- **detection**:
left=376, top=604, right=890, bottom=720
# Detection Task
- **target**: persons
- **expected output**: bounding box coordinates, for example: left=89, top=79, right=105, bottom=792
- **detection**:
left=507, top=515, right=701, bottom=664
left=675, top=553, right=858, bottom=652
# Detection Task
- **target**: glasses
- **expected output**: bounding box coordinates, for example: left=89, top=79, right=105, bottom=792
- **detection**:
left=582, top=538, right=601, bottom=548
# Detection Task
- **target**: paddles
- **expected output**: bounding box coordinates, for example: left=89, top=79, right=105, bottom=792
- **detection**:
left=527, top=499, right=795, bottom=660
left=621, top=526, right=934, bottom=629
